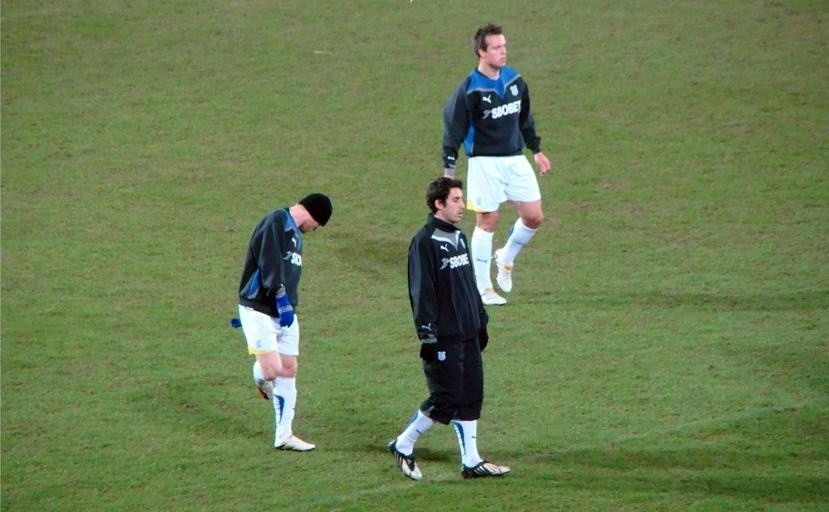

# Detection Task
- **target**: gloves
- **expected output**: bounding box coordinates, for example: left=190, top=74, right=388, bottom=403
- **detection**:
left=478, top=328, right=489, bottom=352
left=419, top=339, right=442, bottom=365
left=275, top=293, right=294, bottom=328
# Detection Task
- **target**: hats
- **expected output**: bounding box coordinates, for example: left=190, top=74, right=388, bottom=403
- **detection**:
left=298, top=193, right=333, bottom=227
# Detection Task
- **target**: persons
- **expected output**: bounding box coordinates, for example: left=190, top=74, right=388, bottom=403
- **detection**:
left=237, top=192, right=333, bottom=454
left=390, top=179, right=513, bottom=481
left=441, top=20, right=553, bottom=306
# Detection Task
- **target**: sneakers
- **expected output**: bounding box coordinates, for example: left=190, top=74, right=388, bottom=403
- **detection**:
left=459, top=460, right=512, bottom=480
left=387, top=437, right=423, bottom=481
left=494, top=248, right=513, bottom=293
left=273, top=433, right=317, bottom=451
left=476, top=287, right=508, bottom=306
left=254, top=376, right=275, bottom=400
